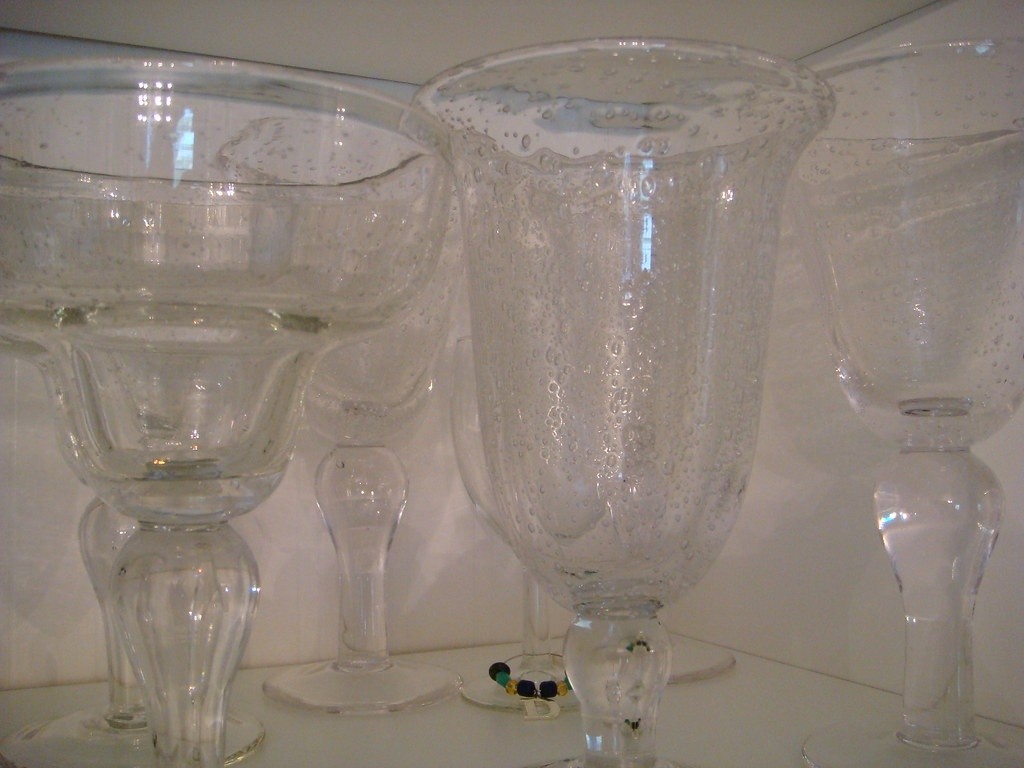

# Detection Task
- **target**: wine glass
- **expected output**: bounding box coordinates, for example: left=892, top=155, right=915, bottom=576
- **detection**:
left=795, top=38, right=1024, bottom=768
left=0, top=324, right=266, bottom=768
left=0, top=54, right=454, bottom=768
left=410, top=34, right=836, bottom=768
left=216, top=115, right=461, bottom=716
left=448, top=335, right=613, bottom=707
left=585, top=613, right=738, bottom=685
